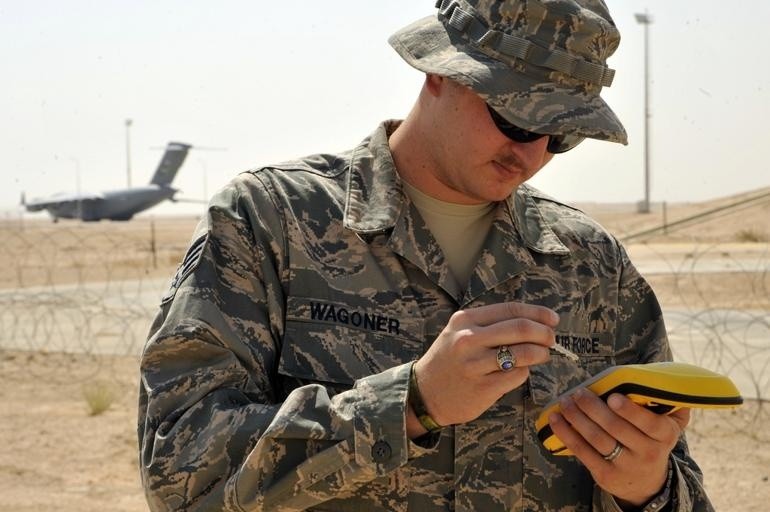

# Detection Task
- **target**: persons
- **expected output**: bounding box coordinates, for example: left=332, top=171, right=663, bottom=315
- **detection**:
left=138, top=0, right=716, bottom=511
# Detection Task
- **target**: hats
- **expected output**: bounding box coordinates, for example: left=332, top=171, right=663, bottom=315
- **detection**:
left=386, top=2, right=632, bottom=148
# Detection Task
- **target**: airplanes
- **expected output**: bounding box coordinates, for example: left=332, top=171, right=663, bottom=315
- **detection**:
left=21, top=144, right=190, bottom=222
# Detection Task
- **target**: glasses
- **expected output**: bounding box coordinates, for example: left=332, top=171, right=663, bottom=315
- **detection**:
left=483, top=97, right=592, bottom=155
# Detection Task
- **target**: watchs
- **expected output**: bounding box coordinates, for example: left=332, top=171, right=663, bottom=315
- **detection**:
left=644, top=461, right=674, bottom=512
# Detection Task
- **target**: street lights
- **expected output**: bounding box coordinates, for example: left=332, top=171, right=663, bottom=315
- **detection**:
left=637, top=13, right=650, bottom=214
left=125, top=119, right=132, bottom=187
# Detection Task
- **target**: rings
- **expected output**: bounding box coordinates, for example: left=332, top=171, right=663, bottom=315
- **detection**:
left=495, top=344, right=517, bottom=372
left=604, top=442, right=623, bottom=463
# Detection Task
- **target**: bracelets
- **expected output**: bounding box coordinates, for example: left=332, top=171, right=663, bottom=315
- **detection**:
left=405, top=363, right=447, bottom=435
